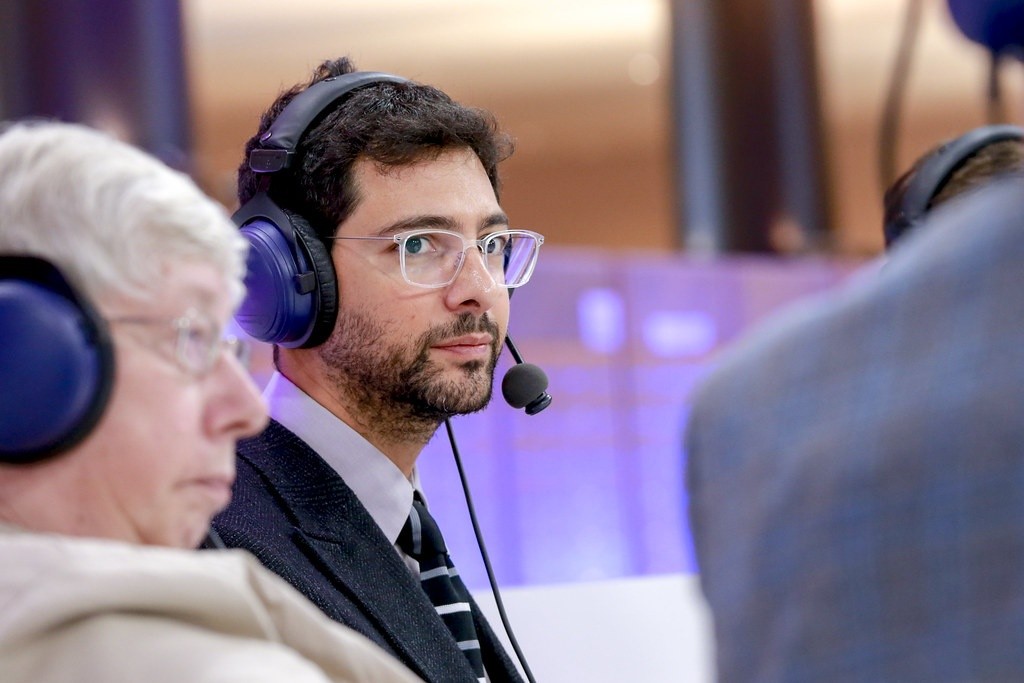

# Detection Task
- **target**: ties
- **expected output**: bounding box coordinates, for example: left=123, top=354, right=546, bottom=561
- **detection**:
left=394, top=492, right=485, bottom=683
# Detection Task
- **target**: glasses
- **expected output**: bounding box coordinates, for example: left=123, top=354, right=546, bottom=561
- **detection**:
left=104, top=314, right=246, bottom=373
left=317, top=230, right=545, bottom=290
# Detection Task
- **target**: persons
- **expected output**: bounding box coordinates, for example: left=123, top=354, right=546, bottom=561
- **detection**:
left=196, top=55, right=544, bottom=683
left=684, top=176, right=1024, bottom=683
left=881, top=122, right=1024, bottom=250
left=0, top=118, right=421, bottom=683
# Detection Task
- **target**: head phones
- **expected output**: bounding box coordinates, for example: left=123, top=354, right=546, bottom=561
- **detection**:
left=0, top=249, right=121, bottom=464
left=228, top=70, right=521, bottom=349
left=942, top=0, right=1024, bottom=65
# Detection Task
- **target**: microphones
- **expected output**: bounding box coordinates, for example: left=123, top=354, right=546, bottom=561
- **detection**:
left=501, top=334, right=553, bottom=415
